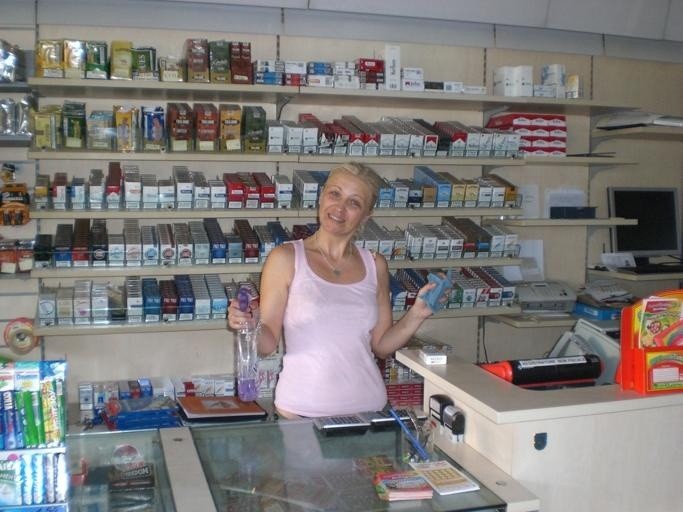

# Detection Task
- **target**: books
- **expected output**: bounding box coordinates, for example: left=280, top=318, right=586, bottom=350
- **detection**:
left=407, top=460, right=480, bottom=498
left=175, top=395, right=269, bottom=428
left=371, top=469, right=432, bottom=503
left=573, top=276, right=633, bottom=311
left=636, top=295, right=682, bottom=346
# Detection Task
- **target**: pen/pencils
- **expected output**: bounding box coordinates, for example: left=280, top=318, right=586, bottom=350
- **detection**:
left=390, top=408, right=436, bottom=463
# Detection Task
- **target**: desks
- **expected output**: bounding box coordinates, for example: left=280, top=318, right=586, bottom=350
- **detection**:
left=587, top=268, right=683, bottom=302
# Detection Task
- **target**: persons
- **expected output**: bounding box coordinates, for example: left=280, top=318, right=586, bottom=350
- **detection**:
left=226, top=161, right=453, bottom=423
left=242, top=418, right=399, bottom=512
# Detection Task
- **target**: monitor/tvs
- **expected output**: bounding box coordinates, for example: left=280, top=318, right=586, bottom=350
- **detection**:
left=607, top=186, right=682, bottom=266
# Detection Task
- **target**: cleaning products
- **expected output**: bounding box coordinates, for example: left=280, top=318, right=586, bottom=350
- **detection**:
left=235, top=283, right=261, bottom=401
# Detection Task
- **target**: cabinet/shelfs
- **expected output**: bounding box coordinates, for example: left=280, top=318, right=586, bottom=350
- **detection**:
left=30, top=77, right=526, bottom=336
left=489, top=95, right=637, bottom=329
left=0, top=81, right=33, bottom=280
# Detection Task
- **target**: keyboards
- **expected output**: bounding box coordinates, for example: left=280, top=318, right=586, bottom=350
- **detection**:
left=617, top=266, right=683, bottom=275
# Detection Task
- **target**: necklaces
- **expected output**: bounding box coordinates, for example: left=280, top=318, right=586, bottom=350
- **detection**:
left=313, top=230, right=353, bottom=276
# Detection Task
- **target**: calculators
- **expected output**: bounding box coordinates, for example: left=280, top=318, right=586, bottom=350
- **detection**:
left=313, top=409, right=415, bottom=437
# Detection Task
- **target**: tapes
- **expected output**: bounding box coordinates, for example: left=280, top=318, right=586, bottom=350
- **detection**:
left=3, top=317, right=41, bottom=355
